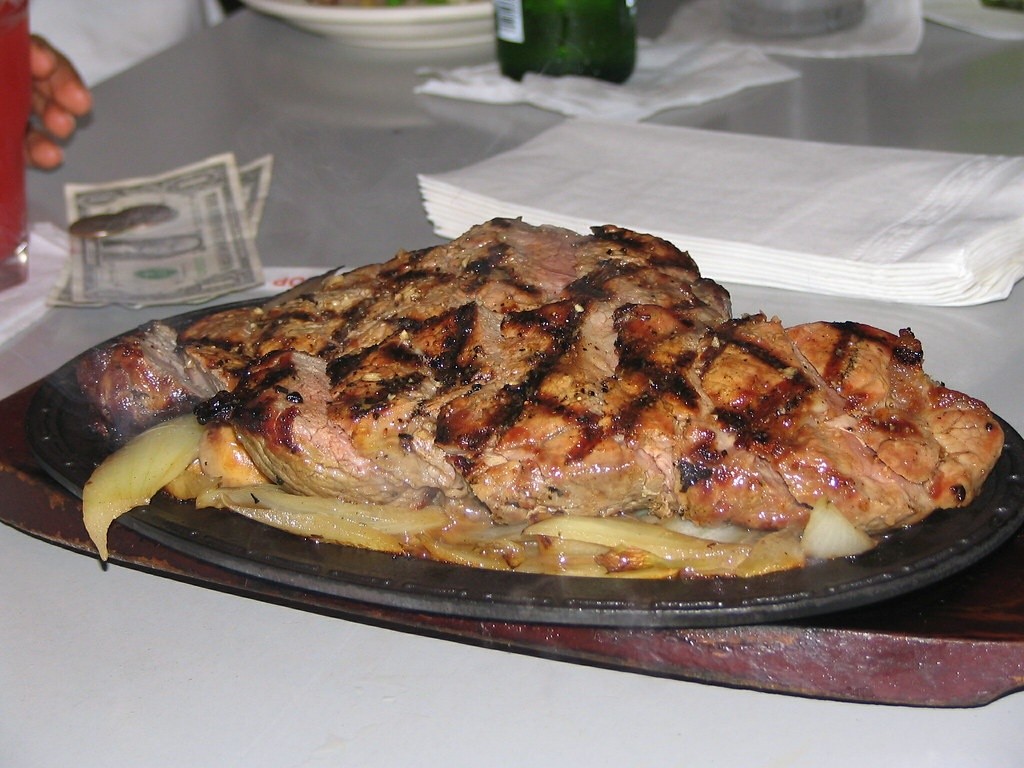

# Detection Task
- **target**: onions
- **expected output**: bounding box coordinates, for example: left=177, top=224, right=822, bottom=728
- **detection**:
left=83, top=412, right=876, bottom=576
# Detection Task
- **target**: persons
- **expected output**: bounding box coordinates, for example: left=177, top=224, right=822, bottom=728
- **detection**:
left=25, top=34, right=92, bottom=169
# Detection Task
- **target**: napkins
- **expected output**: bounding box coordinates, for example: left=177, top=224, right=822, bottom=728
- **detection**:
left=413, top=0, right=1024, bottom=307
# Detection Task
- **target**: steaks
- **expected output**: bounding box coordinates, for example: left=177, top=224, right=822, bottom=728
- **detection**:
left=71, top=214, right=1006, bottom=535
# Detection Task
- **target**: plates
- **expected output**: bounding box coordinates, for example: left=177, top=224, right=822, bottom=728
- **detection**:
left=24, top=293, right=1024, bottom=631
left=241, top=0, right=496, bottom=51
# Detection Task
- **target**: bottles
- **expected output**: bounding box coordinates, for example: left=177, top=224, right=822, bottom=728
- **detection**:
left=492, top=0, right=639, bottom=84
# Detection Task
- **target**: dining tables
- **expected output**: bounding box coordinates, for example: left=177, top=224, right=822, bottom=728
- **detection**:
left=0, top=8, right=1023, bottom=768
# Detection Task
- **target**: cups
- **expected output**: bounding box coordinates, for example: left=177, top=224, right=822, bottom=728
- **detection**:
left=0, top=0, right=32, bottom=290
